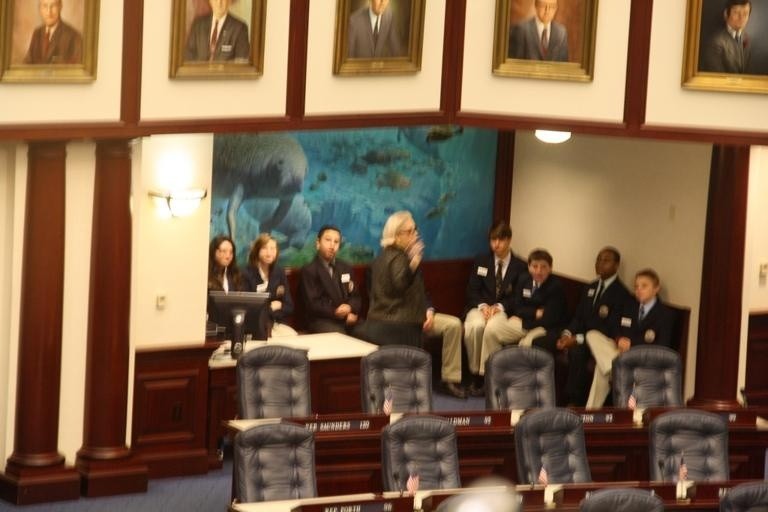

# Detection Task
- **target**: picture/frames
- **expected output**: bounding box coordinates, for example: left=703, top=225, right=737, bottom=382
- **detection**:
left=167, top=0, right=267, bottom=81
left=490, top=1, right=599, bottom=83
left=331, top=1, right=427, bottom=76
left=682, top=0, right=768, bottom=95
left=1, top=0, right=102, bottom=85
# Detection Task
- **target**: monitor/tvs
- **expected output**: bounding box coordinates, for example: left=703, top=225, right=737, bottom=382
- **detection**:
left=207, top=294, right=271, bottom=359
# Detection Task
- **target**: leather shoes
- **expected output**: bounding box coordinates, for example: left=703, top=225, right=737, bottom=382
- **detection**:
left=471, top=386, right=486, bottom=396
left=440, top=382, right=468, bottom=399
left=468, top=382, right=477, bottom=393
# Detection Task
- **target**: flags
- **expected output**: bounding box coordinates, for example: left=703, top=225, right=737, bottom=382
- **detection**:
left=680, top=454, right=688, bottom=482
left=407, top=465, right=420, bottom=498
left=539, top=462, right=549, bottom=486
left=383, top=388, right=392, bottom=416
left=627, top=383, right=636, bottom=410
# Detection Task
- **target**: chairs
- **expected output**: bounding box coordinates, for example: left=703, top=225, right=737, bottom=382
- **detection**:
left=646, top=410, right=730, bottom=485
left=359, top=345, right=434, bottom=414
left=235, top=343, right=314, bottom=422
left=579, top=485, right=668, bottom=512
left=612, top=343, right=685, bottom=408
left=716, top=479, right=767, bottom=511
left=482, top=344, right=560, bottom=413
left=512, top=407, right=591, bottom=487
left=379, top=418, right=463, bottom=490
left=228, top=423, right=317, bottom=502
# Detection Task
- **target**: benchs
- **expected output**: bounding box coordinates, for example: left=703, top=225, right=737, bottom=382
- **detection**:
left=232, top=256, right=689, bottom=394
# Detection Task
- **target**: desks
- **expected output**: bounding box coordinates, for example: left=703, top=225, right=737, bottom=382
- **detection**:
left=210, top=329, right=381, bottom=454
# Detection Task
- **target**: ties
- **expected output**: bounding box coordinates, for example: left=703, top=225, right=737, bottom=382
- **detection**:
left=541, top=26, right=548, bottom=53
left=736, top=34, right=741, bottom=46
left=42, top=28, right=49, bottom=56
left=532, top=280, right=539, bottom=294
left=373, top=15, right=378, bottom=45
left=329, top=264, right=343, bottom=299
left=593, top=281, right=604, bottom=308
left=495, top=261, right=503, bottom=299
left=210, top=19, right=219, bottom=60
left=640, top=305, right=644, bottom=322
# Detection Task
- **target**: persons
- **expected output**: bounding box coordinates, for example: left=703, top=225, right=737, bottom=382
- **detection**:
left=705, top=1, right=756, bottom=72
left=297, top=225, right=366, bottom=337
left=182, top=0, right=249, bottom=63
left=470, top=250, right=571, bottom=394
left=367, top=210, right=465, bottom=398
left=585, top=269, right=666, bottom=410
left=209, top=235, right=255, bottom=330
left=510, top=0, right=567, bottom=61
left=243, top=234, right=297, bottom=337
left=342, top=1, right=411, bottom=61
left=464, top=221, right=528, bottom=395
left=23, top=1, right=84, bottom=65
left=555, top=247, right=632, bottom=406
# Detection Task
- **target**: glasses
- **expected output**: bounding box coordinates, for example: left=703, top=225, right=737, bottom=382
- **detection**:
left=398, top=226, right=417, bottom=235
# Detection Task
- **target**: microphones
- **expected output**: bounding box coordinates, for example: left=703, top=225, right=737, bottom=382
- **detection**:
left=740, top=390, right=746, bottom=408
left=631, top=377, right=636, bottom=398
left=658, top=459, right=665, bottom=484
left=370, top=394, right=379, bottom=416
left=495, top=388, right=502, bottom=412
left=393, top=471, right=403, bottom=497
left=524, top=466, right=534, bottom=490
